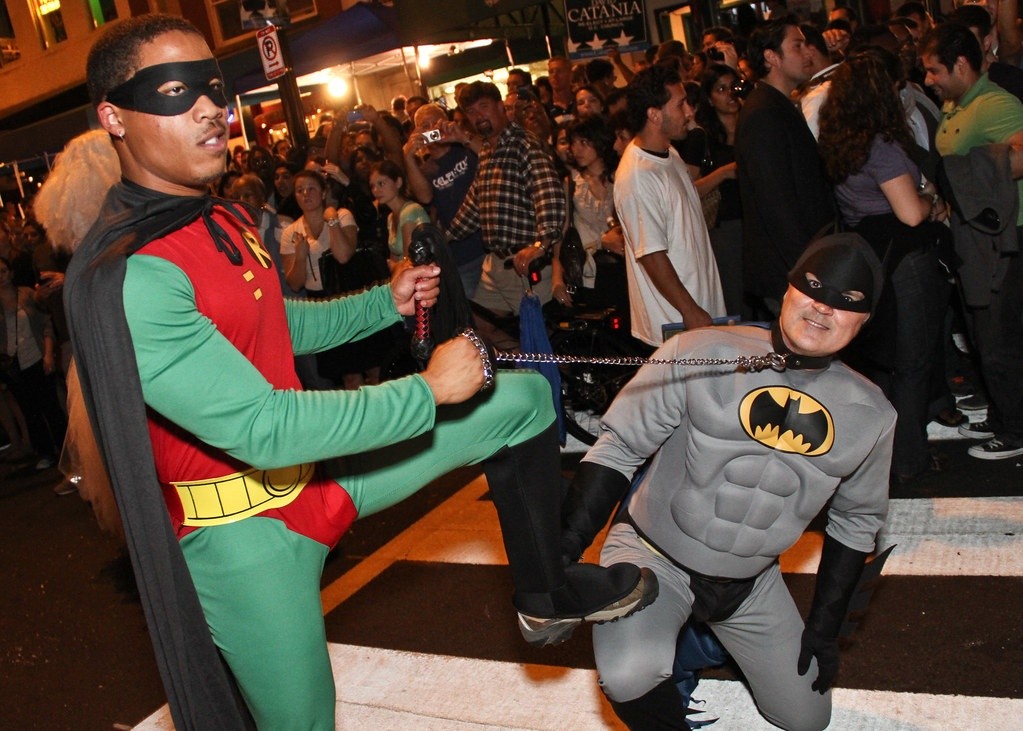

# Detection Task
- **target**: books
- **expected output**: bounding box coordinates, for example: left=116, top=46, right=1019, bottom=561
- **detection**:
left=662, top=314, right=741, bottom=344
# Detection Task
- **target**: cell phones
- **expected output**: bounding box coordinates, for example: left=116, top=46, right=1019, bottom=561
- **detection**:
left=346, top=109, right=363, bottom=121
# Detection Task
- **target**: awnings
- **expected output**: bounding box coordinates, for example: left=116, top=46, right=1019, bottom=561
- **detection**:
left=218, top=28, right=322, bottom=108
left=0, top=101, right=102, bottom=164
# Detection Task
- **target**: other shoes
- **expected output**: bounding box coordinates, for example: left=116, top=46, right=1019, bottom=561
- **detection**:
left=35, top=457, right=55, bottom=471
left=955, top=392, right=990, bottom=411
left=53, top=478, right=79, bottom=496
left=931, top=405, right=969, bottom=427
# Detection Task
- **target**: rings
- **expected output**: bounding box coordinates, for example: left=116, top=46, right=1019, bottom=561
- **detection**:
left=621, top=247, right=625, bottom=252
left=522, top=263, right=526, bottom=266
left=561, top=299, right=565, bottom=302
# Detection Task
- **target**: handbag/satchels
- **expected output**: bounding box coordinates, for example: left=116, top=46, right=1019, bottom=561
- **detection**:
left=517, top=296, right=566, bottom=446
left=687, top=127, right=721, bottom=231
left=558, top=175, right=586, bottom=290
left=319, top=242, right=384, bottom=297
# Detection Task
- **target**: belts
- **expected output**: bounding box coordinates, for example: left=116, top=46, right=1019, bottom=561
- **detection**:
left=494, top=245, right=528, bottom=259
left=168, top=462, right=316, bottom=527
left=594, top=252, right=621, bottom=263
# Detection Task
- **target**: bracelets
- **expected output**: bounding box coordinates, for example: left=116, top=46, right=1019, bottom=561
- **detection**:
left=462, top=130, right=474, bottom=146
left=920, top=192, right=934, bottom=202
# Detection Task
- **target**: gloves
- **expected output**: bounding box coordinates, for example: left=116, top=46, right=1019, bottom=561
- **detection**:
left=559, top=461, right=630, bottom=562
left=797, top=531, right=871, bottom=694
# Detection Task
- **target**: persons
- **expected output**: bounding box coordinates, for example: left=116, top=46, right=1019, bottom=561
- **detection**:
left=0, top=0, right=1023, bottom=565
left=521, top=234, right=897, bottom=731
left=62, top=13, right=658, bottom=731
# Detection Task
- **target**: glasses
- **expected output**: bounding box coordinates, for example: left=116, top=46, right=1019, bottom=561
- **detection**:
left=235, top=192, right=253, bottom=200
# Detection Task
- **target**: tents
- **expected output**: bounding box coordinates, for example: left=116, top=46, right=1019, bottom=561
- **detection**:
left=233, top=0, right=413, bottom=150
left=400, top=0, right=568, bottom=105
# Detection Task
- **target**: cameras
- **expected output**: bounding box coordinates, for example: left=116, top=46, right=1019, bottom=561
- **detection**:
left=422, top=129, right=441, bottom=144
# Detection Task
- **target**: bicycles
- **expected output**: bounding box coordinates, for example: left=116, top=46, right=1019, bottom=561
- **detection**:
left=466, top=257, right=651, bottom=446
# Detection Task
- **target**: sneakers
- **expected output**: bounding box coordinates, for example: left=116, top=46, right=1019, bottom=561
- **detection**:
left=513, top=567, right=660, bottom=651
left=966, top=435, right=1023, bottom=460
left=957, top=420, right=996, bottom=439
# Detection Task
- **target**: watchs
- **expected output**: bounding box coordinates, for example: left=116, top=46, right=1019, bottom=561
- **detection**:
left=328, top=218, right=339, bottom=226
left=534, top=241, right=547, bottom=251
